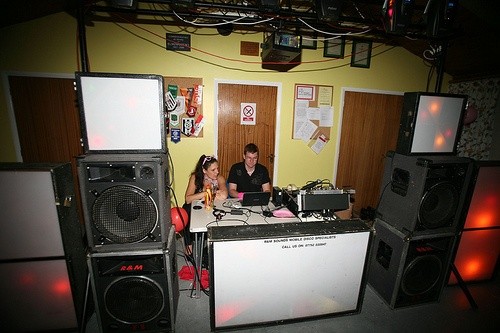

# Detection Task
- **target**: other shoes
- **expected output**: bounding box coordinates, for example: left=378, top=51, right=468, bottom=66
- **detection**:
left=186, top=243, right=193, bottom=261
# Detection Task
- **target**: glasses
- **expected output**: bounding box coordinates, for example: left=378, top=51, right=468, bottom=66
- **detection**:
left=202, top=156, right=213, bottom=165
left=247, top=157, right=258, bottom=161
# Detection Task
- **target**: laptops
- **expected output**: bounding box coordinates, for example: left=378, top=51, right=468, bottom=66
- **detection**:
left=240, top=192, right=271, bottom=206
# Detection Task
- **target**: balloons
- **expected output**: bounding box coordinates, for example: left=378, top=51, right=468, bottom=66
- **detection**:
left=171, top=207, right=189, bottom=232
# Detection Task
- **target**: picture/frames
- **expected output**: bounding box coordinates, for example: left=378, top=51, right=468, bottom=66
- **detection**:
left=350, top=37, right=373, bottom=68
left=324, top=37, right=345, bottom=59
left=301, top=35, right=318, bottom=50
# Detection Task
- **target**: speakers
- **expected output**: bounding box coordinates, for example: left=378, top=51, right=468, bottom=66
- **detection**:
left=76, top=153, right=180, bottom=333
left=366, top=150, right=480, bottom=309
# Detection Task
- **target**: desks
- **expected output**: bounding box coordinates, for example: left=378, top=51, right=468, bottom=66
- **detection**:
left=189, top=196, right=337, bottom=299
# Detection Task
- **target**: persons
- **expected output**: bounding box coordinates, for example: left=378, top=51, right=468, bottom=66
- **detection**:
left=183, top=154, right=228, bottom=261
left=228, top=143, right=271, bottom=198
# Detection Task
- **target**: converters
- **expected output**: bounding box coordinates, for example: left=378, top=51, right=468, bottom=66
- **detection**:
left=231, top=210, right=243, bottom=215
